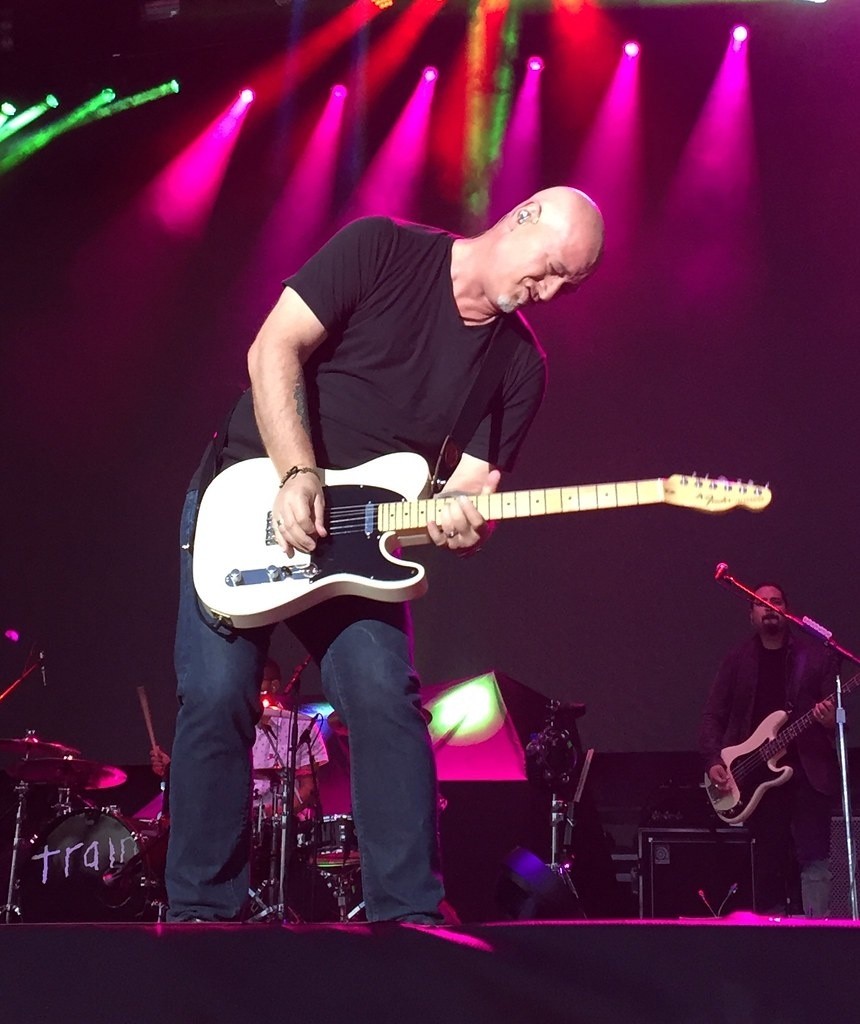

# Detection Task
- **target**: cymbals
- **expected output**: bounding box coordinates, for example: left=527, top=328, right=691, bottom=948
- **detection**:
left=327, top=709, right=350, bottom=736
left=253, top=765, right=312, bottom=780
left=1, top=735, right=80, bottom=757
left=6, top=754, right=130, bottom=790
left=420, top=708, right=433, bottom=726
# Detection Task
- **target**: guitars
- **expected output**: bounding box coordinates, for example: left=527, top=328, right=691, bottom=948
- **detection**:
left=703, top=672, right=860, bottom=824
left=190, top=451, right=774, bottom=631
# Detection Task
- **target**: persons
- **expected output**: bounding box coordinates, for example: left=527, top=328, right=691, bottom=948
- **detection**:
left=150, top=658, right=329, bottom=837
left=699, top=582, right=837, bottom=918
left=166, top=185, right=605, bottom=922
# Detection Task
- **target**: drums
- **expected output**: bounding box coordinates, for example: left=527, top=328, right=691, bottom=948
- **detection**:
left=28, top=805, right=167, bottom=922
left=312, top=813, right=359, bottom=856
left=315, top=858, right=366, bottom=923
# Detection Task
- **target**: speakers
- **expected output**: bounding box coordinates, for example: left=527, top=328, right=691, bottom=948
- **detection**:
left=636, top=828, right=756, bottom=919
left=786, top=809, right=860, bottom=918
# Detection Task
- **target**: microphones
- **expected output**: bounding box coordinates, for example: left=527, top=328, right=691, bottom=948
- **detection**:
left=296, top=713, right=318, bottom=750
left=714, top=562, right=728, bottom=581
left=39, top=648, right=48, bottom=686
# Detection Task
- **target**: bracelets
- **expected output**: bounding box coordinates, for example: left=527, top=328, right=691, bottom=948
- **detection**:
left=279, top=466, right=321, bottom=488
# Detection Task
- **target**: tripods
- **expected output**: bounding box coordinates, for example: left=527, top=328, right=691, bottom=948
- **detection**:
left=242, top=655, right=312, bottom=925
left=0, top=728, right=37, bottom=924
left=521, top=697, right=589, bottom=920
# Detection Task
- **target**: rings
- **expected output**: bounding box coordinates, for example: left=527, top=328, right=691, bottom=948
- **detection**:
left=821, top=715, right=824, bottom=719
left=715, top=784, right=718, bottom=788
left=447, top=530, right=458, bottom=538
left=276, top=519, right=284, bottom=526
